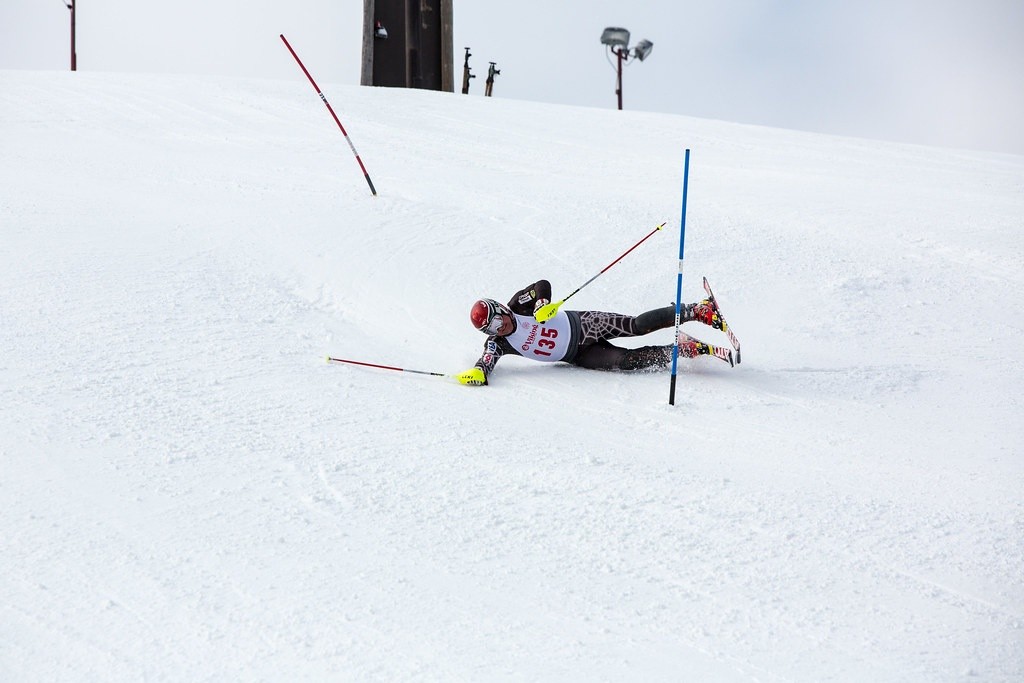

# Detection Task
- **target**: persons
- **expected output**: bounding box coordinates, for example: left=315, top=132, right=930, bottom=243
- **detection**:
left=458, top=280, right=724, bottom=386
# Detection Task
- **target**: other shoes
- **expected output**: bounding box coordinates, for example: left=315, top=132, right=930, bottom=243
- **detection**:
left=671, top=342, right=697, bottom=358
left=695, top=299, right=719, bottom=330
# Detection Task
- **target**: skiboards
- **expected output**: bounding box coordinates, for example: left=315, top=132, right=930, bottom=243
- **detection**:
left=678, top=276, right=741, bottom=368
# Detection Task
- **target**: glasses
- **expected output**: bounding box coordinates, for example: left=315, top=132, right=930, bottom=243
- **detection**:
left=482, top=298, right=504, bottom=336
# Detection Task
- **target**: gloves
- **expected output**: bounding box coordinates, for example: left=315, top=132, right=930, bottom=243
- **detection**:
left=466, top=366, right=488, bottom=386
left=533, top=299, right=550, bottom=324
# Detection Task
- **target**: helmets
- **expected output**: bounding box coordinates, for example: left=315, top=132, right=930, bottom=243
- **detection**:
left=470, top=298, right=512, bottom=337
